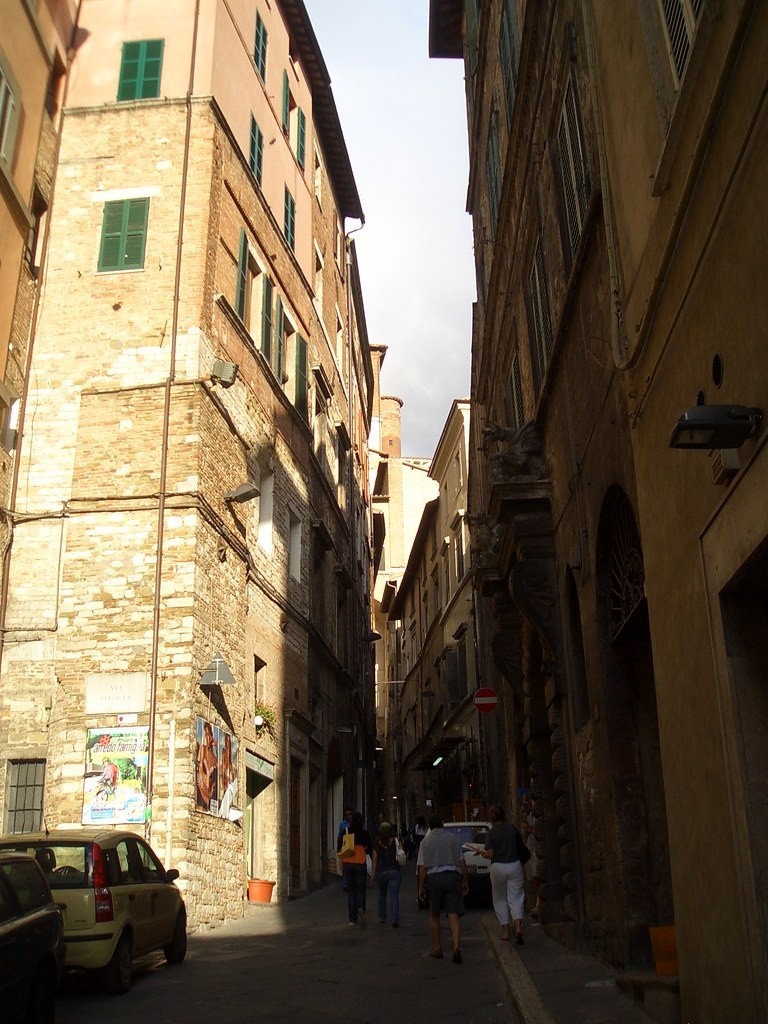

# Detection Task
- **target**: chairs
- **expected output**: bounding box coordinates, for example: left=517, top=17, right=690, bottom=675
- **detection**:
left=35, top=848, right=56, bottom=872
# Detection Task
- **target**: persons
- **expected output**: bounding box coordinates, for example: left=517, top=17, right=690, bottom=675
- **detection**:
left=372, top=813, right=402, bottom=928
left=198, top=721, right=235, bottom=813
left=401, top=814, right=427, bottom=854
left=416, top=814, right=469, bottom=963
left=482, top=805, right=531, bottom=944
left=337, top=808, right=368, bottom=852
left=336, top=812, right=374, bottom=926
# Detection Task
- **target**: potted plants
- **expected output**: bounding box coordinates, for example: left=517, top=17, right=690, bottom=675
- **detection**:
left=254, top=701, right=277, bottom=726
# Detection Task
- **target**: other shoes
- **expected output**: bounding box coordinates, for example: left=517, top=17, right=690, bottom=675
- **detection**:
left=517, top=931, right=524, bottom=944
left=392, top=922, right=399, bottom=928
left=350, top=922, right=355, bottom=926
left=454, top=950, right=461, bottom=962
left=429, top=951, right=443, bottom=958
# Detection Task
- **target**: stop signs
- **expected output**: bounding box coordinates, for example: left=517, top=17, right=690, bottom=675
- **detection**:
left=473, top=686, right=499, bottom=713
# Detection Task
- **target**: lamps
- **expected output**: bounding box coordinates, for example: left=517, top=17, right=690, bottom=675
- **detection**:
left=482, top=420, right=517, bottom=442
left=224, top=483, right=261, bottom=502
left=212, top=359, right=238, bottom=384
left=669, top=404, right=764, bottom=449
left=364, top=633, right=382, bottom=642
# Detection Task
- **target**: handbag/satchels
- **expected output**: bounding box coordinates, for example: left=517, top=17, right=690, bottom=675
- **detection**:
left=394, top=837, right=406, bottom=865
left=337, top=827, right=355, bottom=858
left=513, top=824, right=531, bottom=866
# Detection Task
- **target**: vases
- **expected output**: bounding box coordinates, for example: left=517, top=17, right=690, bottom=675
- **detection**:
left=248, top=878, right=276, bottom=903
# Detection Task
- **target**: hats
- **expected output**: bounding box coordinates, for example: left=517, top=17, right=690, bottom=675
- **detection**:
left=379, top=822, right=392, bottom=831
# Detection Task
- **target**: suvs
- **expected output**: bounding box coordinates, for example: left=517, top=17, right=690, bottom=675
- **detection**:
left=417, top=823, right=498, bottom=900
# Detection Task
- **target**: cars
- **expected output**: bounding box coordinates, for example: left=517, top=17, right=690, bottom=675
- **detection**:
left=0, top=850, right=67, bottom=1024
left=0, top=828, right=187, bottom=997
